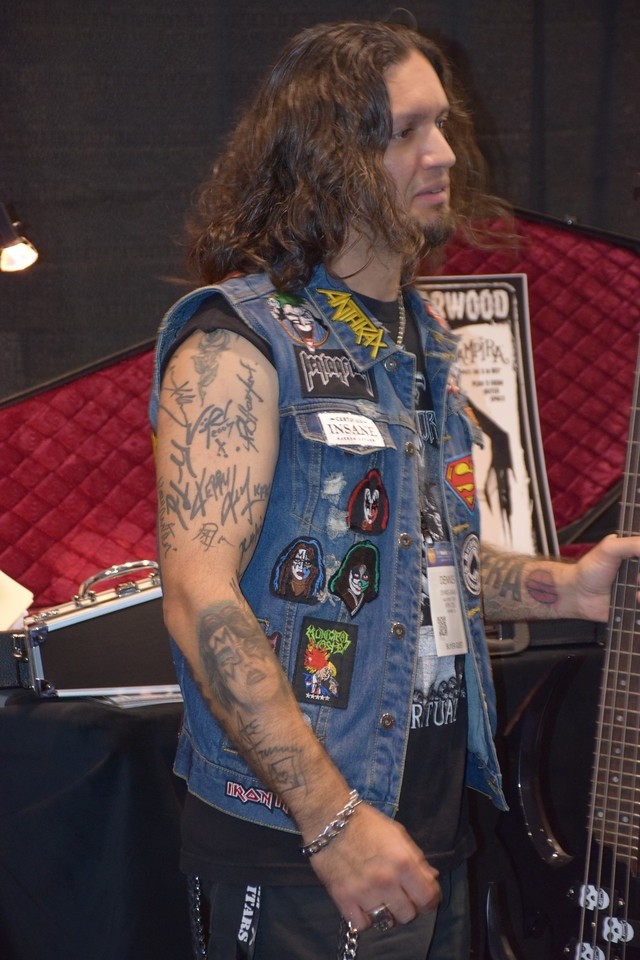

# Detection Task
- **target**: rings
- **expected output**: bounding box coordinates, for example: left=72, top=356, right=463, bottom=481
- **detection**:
left=364, top=904, right=397, bottom=940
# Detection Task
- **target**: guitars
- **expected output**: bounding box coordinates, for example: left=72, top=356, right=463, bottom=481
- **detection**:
left=559, top=347, right=640, bottom=960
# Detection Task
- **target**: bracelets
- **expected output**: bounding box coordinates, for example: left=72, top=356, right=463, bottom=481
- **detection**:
left=294, top=784, right=365, bottom=860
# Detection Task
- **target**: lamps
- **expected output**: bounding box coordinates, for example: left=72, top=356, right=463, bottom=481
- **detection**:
left=0, top=202, right=38, bottom=274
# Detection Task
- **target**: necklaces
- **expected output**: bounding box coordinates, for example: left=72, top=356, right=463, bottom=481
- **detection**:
left=394, top=284, right=408, bottom=345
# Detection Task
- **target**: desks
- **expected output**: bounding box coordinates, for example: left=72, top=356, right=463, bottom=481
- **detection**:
left=0, top=647, right=603, bottom=960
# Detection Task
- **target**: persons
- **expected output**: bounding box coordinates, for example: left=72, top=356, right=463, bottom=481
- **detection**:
left=148, top=9, right=640, bottom=960
